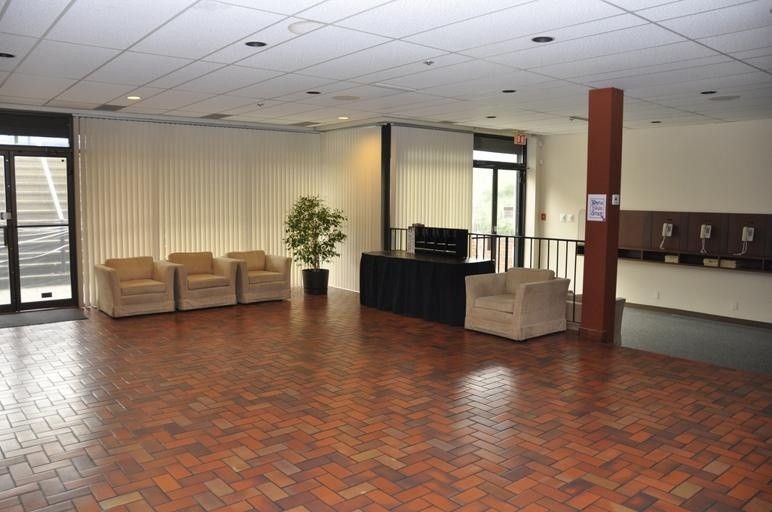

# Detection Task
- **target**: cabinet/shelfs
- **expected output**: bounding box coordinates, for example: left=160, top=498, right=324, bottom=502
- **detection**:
left=618, top=212, right=771, bottom=274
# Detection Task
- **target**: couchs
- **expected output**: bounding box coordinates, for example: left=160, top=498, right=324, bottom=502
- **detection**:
left=93, top=255, right=176, bottom=320
left=463, top=266, right=571, bottom=343
left=165, top=251, right=237, bottom=314
left=566, top=293, right=626, bottom=347
left=225, top=249, right=292, bottom=305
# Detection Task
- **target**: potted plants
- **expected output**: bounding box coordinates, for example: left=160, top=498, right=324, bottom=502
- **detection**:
left=281, top=194, right=349, bottom=295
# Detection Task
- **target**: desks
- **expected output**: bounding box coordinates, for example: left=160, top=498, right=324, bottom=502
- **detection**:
left=359, top=249, right=495, bottom=328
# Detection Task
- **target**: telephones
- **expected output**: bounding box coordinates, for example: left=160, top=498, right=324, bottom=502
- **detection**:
left=742, top=227, right=754, bottom=242
left=662, top=223, right=673, bottom=237
left=700, top=224, right=712, bottom=239
